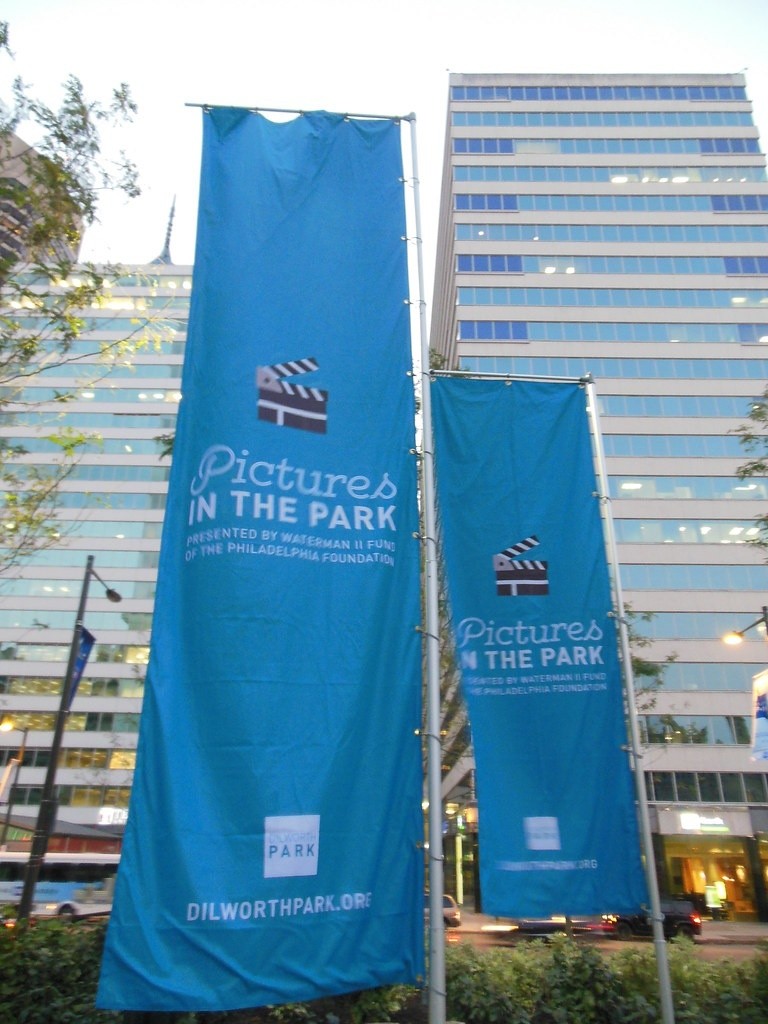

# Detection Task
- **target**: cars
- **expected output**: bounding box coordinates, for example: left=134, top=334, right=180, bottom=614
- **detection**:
left=423, top=893, right=462, bottom=927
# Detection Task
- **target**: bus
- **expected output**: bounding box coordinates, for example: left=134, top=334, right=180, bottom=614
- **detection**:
left=0, top=851, right=122, bottom=920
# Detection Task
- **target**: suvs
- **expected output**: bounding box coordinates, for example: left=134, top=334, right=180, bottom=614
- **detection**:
left=599, top=898, right=700, bottom=944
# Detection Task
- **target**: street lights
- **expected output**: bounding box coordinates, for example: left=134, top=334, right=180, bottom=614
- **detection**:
left=16, top=554, right=124, bottom=929
